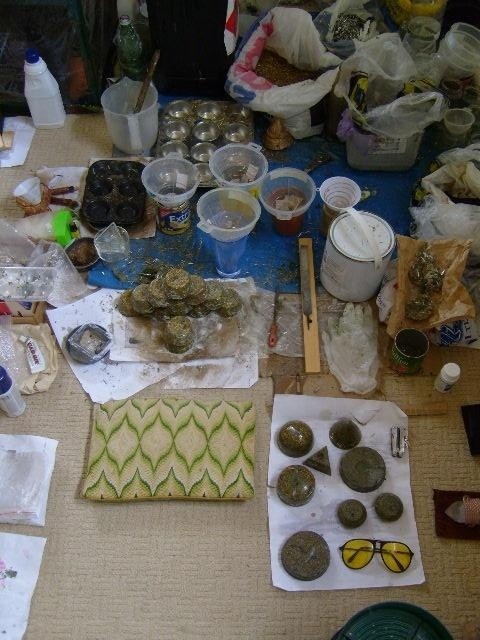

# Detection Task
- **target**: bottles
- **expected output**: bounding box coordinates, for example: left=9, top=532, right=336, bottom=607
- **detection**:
left=23, top=48, right=66, bottom=130
left=434, top=362, right=461, bottom=393
left=113, top=14, right=147, bottom=81
left=0, top=366, right=26, bottom=418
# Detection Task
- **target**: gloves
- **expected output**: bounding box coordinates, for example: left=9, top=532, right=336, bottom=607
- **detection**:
left=321, top=302, right=379, bottom=395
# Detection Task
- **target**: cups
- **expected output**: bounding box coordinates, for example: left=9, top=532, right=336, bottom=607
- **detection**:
left=100, top=76, right=158, bottom=155
